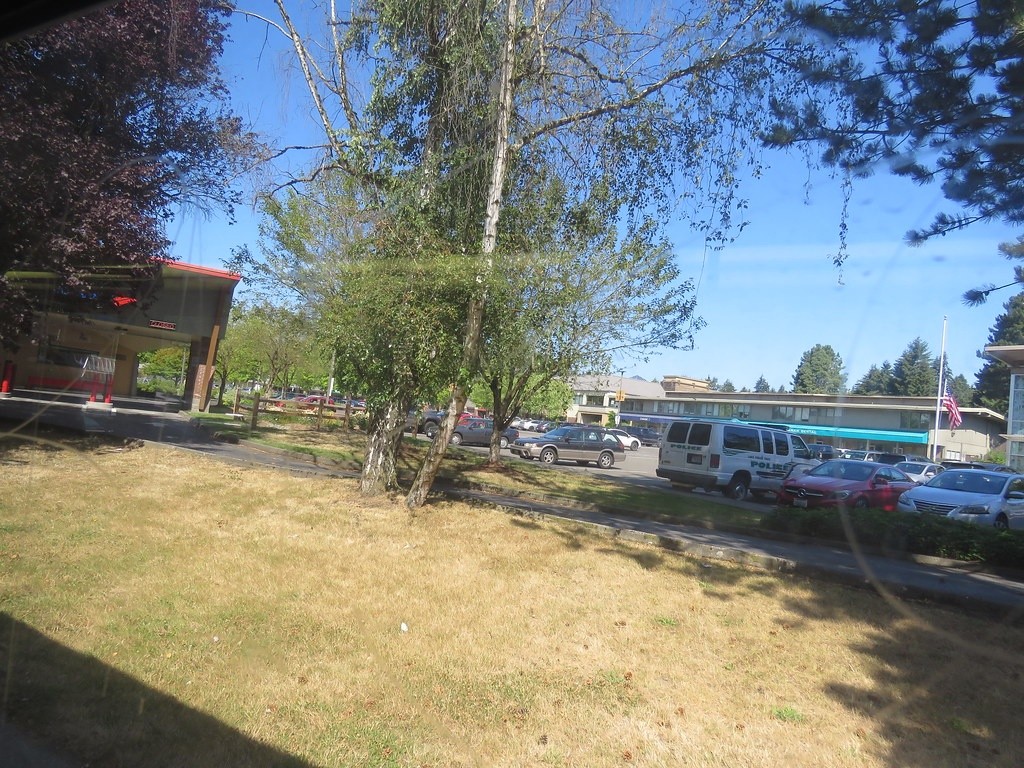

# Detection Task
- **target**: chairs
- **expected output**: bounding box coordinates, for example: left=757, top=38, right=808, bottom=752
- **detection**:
left=962, top=476, right=987, bottom=492
left=843, top=465, right=863, bottom=479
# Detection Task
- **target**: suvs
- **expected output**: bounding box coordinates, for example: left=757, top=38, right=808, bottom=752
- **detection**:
left=509, top=426, right=627, bottom=469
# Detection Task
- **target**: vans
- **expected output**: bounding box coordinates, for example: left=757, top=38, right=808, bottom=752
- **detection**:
left=656, top=419, right=832, bottom=501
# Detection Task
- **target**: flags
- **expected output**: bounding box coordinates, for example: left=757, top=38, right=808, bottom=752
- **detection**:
left=942, top=381, right=963, bottom=439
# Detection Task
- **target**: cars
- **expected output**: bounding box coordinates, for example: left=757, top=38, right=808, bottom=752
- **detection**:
left=777, top=458, right=918, bottom=511
left=805, top=442, right=1022, bottom=486
left=589, top=429, right=641, bottom=451
left=242, top=387, right=252, bottom=392
left=405, top=411, right=485, bottom=439
left=298, top=394, right=336, bottom=413
left=269, top=391, right=367, bottom=407
left=509, top=417, right=584, bottom=433
left=897, top=467, right=1024, bottom=535
left=450, top=418, right=519, bottom=449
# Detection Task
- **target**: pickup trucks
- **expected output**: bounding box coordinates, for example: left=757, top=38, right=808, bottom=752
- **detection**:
left=617, top=425, right=662, bottom=449
